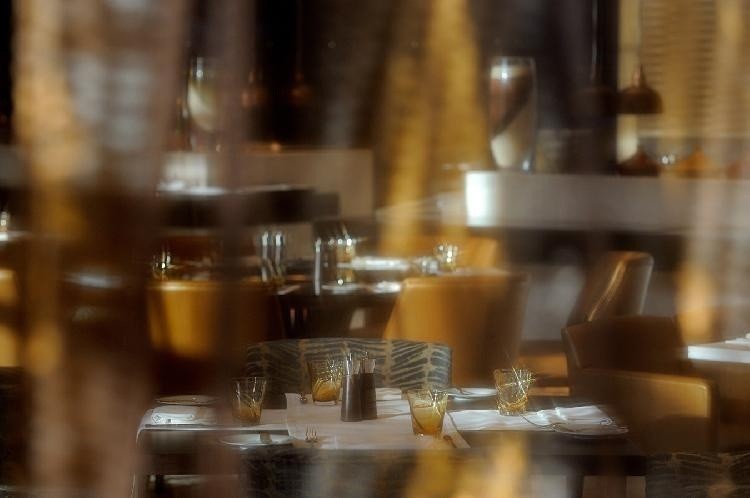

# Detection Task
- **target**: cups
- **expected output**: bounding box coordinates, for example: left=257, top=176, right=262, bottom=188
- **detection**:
left=233, top=375, right=265, bottom=426
left=407, top=387, right=448, bottom=438
left=489, top=55, right=537, bottom=172
left=186, top=56, right=228, bottom=152
left=434, top=244, right=458, bottom=271
left=495, top=367, right=531, bottom=416
left=307, top=355, right=348, bottom=407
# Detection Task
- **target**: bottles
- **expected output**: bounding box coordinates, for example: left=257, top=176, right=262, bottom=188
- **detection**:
left=341, top=355, right=380, bottom=423
left=618, top=62, right=661, bottom=176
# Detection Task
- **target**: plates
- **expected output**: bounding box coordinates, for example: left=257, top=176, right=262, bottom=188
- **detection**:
left=156, top=394, right=216, bottom=405
left=554, top=425, right=629, bottom=436
left=218, top=434, right=294, bottom=447
left=447, top=387, right=495, bottom=398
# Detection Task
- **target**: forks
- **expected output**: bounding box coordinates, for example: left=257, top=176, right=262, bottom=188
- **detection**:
left=305, top=424, right=320, bottom=448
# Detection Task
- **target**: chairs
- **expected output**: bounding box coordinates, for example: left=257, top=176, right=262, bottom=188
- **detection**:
left=513, top=250, right=655, bottom=389
left=233, top=334, right=453, bottom=390
left=382, top=274, right=532, bottom=389
left=560, top=310, right=750, bottom=471
